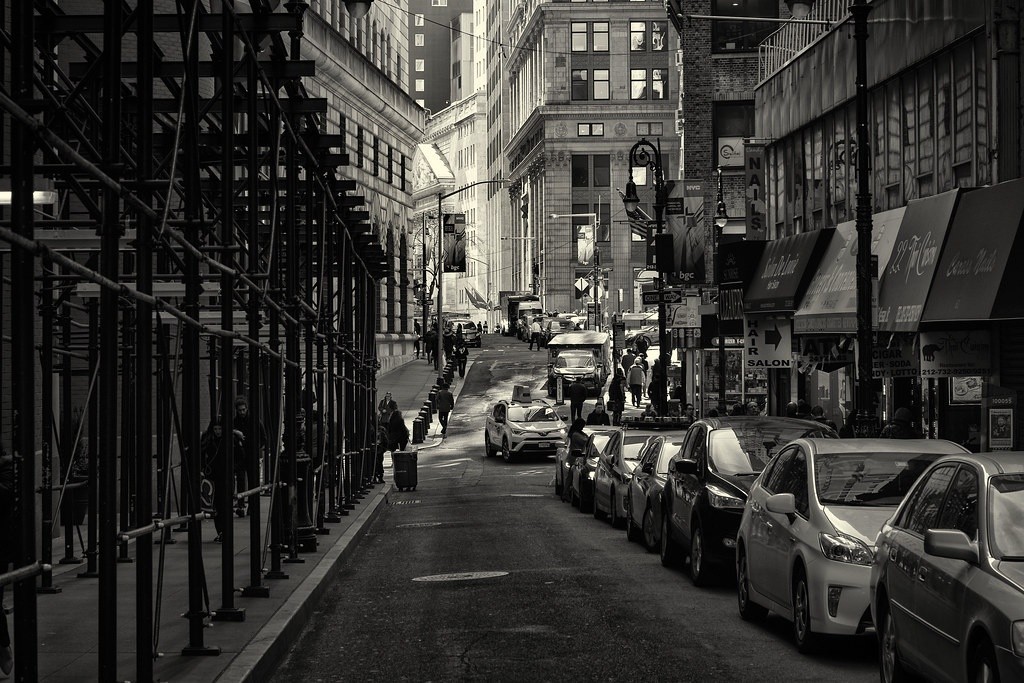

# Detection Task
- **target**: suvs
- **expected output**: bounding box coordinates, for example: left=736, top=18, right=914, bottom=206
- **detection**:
left=548, top=349, right=603, bottom=398
left=485, top=384, right=569, bottom=463
left=659, top=415, right=842, bottom=589
left=446, top=319, right=483, bottom=348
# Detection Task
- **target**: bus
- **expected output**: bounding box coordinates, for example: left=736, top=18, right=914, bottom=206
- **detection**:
left=548, top=329, right=613, bottom=387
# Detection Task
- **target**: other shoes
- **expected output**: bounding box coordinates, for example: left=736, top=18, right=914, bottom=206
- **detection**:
left=214, top=531, right=222, bottom=544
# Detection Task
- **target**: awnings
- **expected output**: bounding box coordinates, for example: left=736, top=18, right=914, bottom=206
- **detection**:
left=741, top=180, right=1024, bottom=332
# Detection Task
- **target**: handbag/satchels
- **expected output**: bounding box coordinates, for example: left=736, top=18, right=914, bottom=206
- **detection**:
left=399, top=439, right=418, bottom=453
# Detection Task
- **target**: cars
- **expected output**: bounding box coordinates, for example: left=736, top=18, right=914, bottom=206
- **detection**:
left=558, top=314, right=587, bottom=332
left=522, top=314, right=576, bottom=349
left=625, top=324, right=660, bottom=348
left=738, top=438, right=973, bottom=657
left=593, top=430, right=661, bottom=528
left=870, top=451, right=1024, bottom=683
left=626, top=430, right=689, bottom=550
left=554, top=424, right=637, bottom=513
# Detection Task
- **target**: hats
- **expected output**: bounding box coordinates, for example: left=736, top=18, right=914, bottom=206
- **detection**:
left=812, top=406, right=824, bottom=416
left=636, top=357, right=642, bottom=362
left=639, top=353, right=644, bottom=357
left=634, top=359, right=640, bottom=363
left=895, top=408, right=912, bottom=422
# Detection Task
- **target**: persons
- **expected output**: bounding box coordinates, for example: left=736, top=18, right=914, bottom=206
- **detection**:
left=562, top=418, right=589, bottom=503
left=684, top=404, right=697, bottom=424
left=388, top=410, right=409, bottom=468
left=648, top=358, right=661, bottom=406
left=379, top=392, right=397, bottom=425
left=528, top=319, right=541, bottom=351
left=232, top=400, right=267, bottom=516
left=568, top=374, right=626, bottom=426
left=414, top=318, right=469, bottom=380
left=708, top=402, right=919, bottom=439
left=436, top=384, right=454, bottom=434
left=477, top=321, right=488, bottom=334
left=545, top=307, right=582, bottom=317
left=621, top=334, right=648, bottom=407
left=372, top=426, right=388, bottom=483
left=202, top=420, right=224, bottom=544
left=574, top=323, right=582, bottom=331
left=496, top=323, right=500, bottom=333
left=0, top=581, right=14, bottom=675
left=992, top=415, right=1011, bottom=438
left=302, top=380, right=344, bottom=501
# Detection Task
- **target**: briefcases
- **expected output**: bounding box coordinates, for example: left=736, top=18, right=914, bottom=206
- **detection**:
left=607, top=399, right=615, bottom=411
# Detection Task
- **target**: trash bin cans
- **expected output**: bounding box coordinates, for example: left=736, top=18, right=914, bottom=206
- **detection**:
left=392, top=451, right=418, bottom=491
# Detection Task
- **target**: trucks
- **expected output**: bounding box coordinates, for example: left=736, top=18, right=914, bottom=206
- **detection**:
left=499, top=294, right=544, bottom=337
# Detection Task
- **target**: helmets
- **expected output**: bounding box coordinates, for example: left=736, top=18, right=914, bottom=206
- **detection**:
left=787, top=402, right=797, bottom=413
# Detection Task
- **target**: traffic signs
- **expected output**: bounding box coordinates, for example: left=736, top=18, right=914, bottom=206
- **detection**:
left=642, top=288, right=682, bottom=305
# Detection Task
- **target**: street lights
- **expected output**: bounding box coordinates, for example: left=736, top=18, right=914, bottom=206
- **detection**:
left=718, top=280, right=746, bottom=416
left=500, top=235, right=538, bottom=295
left=622, top=137, right=668, bottom=411
left=436, top=177, right=519, bottom=381
left=550, top=212, right=600, bottom=326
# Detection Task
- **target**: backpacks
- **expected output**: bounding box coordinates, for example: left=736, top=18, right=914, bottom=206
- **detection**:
left=378, top=426, right=389, bottom=453
